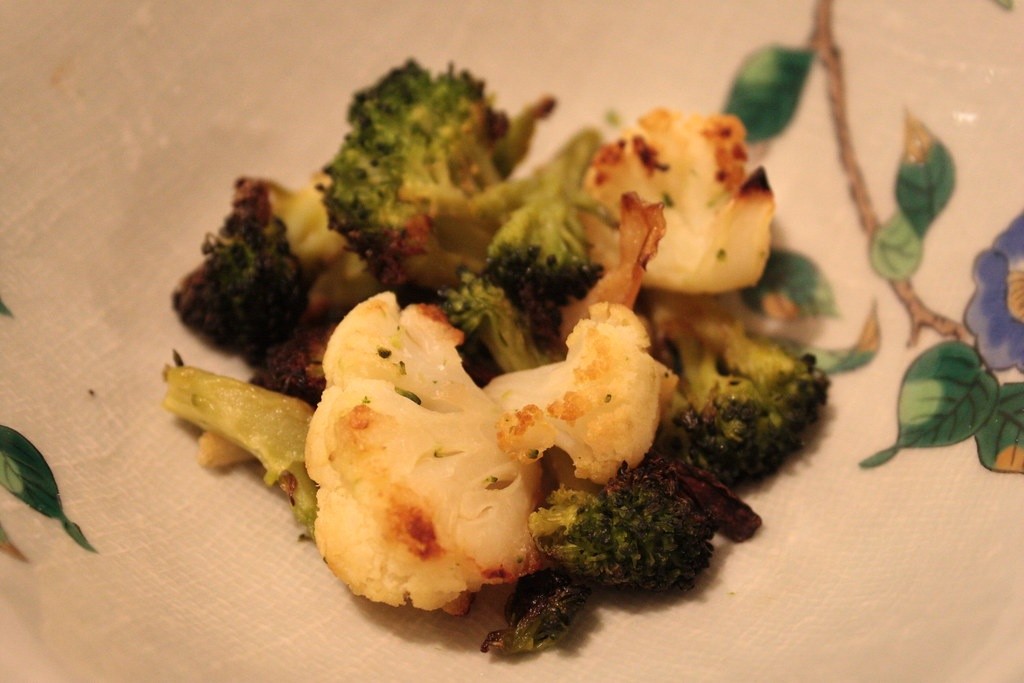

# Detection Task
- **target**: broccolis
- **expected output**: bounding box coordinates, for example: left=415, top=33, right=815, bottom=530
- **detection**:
left=157, top=62, right=831, bottom=654
